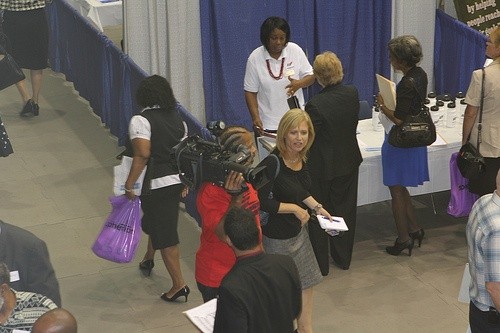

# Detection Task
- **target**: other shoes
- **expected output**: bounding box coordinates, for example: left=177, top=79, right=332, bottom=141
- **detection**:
left=20, top=99, right=34, bottom=114
left=32, top=104, right=39, bottom=115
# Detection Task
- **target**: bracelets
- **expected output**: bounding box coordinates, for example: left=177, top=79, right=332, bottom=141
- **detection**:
left=125, top=187, right=134, bottom=192
left=380, top=104, right=384, bottom=107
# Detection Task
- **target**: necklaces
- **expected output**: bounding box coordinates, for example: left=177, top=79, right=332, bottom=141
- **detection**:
left=287, top=158, right=297, bottom=164
left=266, top=59, right=285, bottom=80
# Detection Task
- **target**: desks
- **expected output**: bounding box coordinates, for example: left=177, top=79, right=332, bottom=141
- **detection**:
left=354, top=98, right=472, bottom=215
left=66, top=0, right=123, bottom=33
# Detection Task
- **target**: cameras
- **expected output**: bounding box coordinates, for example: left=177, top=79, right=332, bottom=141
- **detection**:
left=287, top=95, right=299, bottom=109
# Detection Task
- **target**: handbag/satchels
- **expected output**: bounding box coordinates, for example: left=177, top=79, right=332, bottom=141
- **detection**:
left=0, top=44, right=26, bottom=91
left=447, top=152, right=480, bottom=218
left=91, top=194, right=141, bottom=263
left=248, top=154, right=281, bottom=226
left=457, top=142, right=484, bottom=178
left=388, top=76, right=436, bottom=148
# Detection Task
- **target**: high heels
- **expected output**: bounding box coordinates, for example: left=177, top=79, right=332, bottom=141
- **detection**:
left=409, top=227, right=425, bottom=248
left=161, top=285, right=190, bottom=302
left=139, top=259, right=154, bottom=276
left=386, top=237, right=414, bottom=256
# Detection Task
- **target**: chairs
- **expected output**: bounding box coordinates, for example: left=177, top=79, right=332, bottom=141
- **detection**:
left=359, top=101, right=371, bottom=120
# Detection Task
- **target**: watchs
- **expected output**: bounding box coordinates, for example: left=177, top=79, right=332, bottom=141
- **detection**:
left=313, top=204, right=322, bottom=212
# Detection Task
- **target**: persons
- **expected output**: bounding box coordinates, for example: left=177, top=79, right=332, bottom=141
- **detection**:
left=378, top=36, right=427, bottom=258
left=125, top=74, right=190, bottom=304
left=466, top=169, right=500, bottom=333
left=195, top=15, right=363, bottom=333
left=0, top=0, right=77, bottom=333
left=464, top=27, right=500, bottom=195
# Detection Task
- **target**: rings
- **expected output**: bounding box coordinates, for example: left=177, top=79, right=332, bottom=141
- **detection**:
left=256, top=126, right=259, bottom=129
left=291, top=91, right=294, bottom=93
